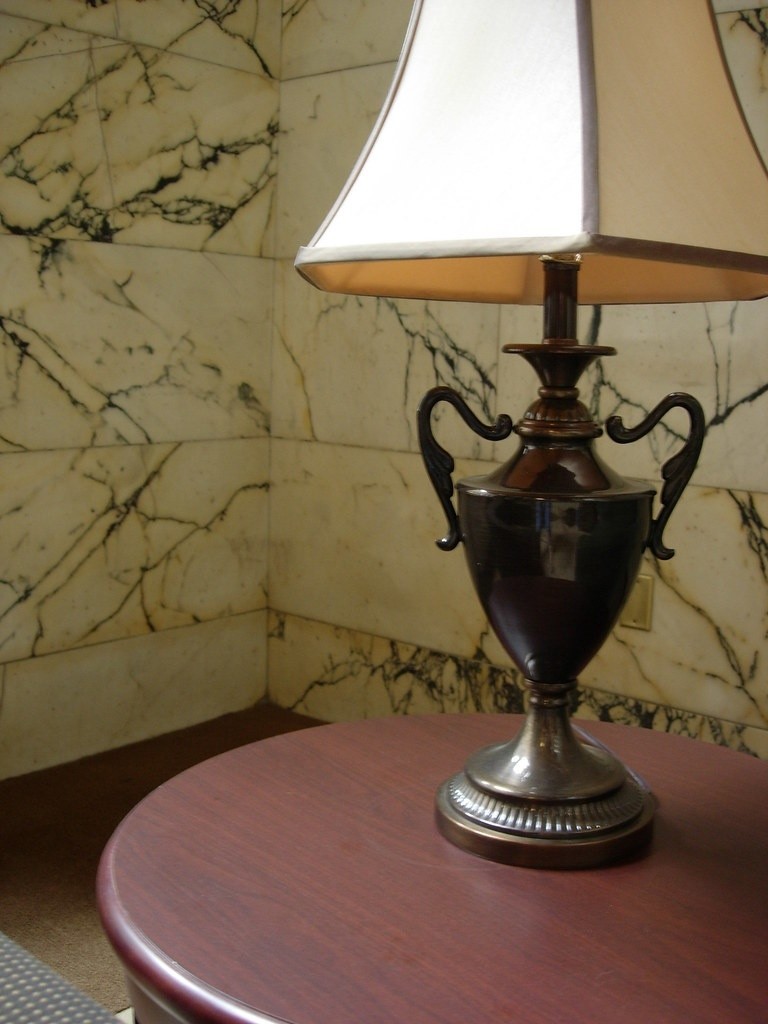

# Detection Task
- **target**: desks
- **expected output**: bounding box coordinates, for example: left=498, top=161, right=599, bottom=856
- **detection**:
left=97, top=702, right=768, bottom=1024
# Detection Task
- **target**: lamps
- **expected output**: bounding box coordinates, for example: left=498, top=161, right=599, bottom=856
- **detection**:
left=289, top=0, right=768, bottom=873
